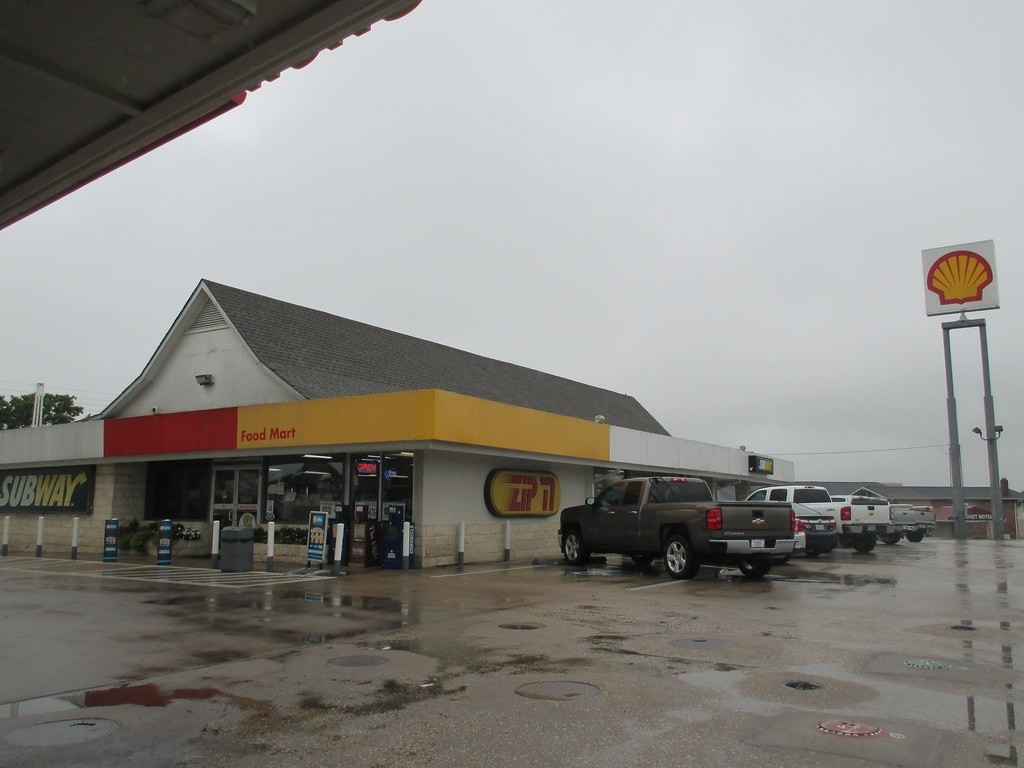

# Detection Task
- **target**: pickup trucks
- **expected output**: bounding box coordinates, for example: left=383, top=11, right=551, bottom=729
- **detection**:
left=829, top=495, right=895, bottom=552
left=557, top=477, right=799, bottom=580
left=877, top=503, right=936, bottom=544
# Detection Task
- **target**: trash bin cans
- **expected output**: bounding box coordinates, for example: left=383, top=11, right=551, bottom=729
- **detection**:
left=221, top=526, right=254, bottom=573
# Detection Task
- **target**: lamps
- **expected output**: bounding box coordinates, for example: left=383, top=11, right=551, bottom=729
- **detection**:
left=195, top=373, right=215, bottom=385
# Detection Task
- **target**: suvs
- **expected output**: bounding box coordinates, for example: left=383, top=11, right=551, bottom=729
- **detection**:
left=745, top=484, right=840, bottom=557
